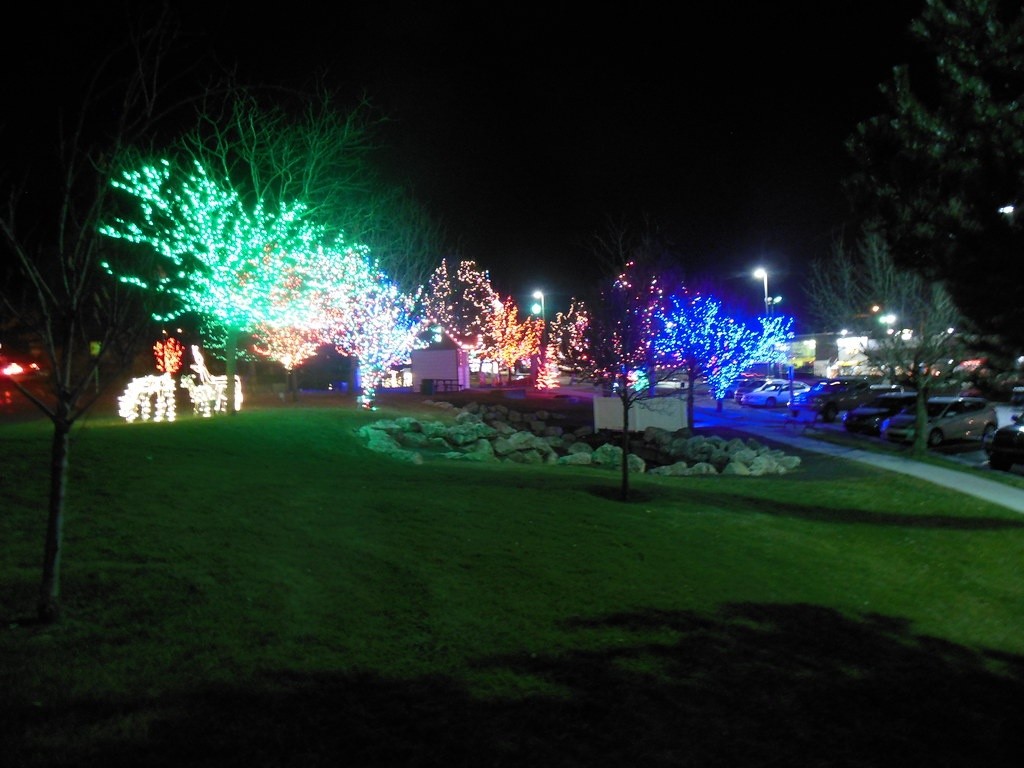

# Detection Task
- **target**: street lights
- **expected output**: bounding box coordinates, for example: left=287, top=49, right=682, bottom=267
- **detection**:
left=533, top=290, right=548, bottom=348
left=767, top=296, right=782, bottom=320
left=753, top=268, right=769, bottom=323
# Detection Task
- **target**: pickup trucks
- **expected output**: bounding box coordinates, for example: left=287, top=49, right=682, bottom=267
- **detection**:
left=787, top=376, right=902, bottom=424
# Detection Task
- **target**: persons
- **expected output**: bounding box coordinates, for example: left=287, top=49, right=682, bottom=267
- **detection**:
left=593, top=373, right=616, bottom=397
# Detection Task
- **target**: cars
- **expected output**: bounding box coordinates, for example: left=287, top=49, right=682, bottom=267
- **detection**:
left=881, top=397, right=999, bottom=448
left=842, top=391, right=928, bottom=437
left=740, top=379, right=812, bottom=408
left=710, top=376, right=767, bottom=404
left=986, top=412, right=1024, bottom=472
